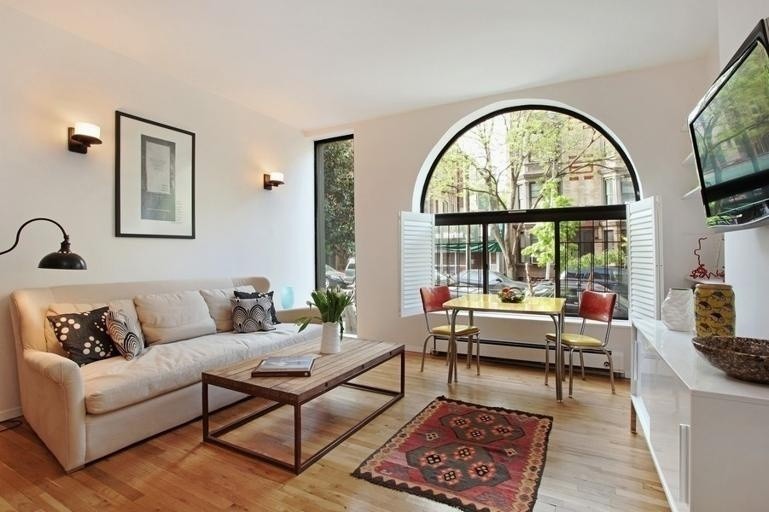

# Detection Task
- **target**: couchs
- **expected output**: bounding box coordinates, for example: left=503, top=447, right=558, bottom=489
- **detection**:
left=10, top=276, right=328, bottom=475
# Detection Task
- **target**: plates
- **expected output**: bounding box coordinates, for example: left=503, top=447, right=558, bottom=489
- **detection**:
left=691, top=337, right=769, bottom=384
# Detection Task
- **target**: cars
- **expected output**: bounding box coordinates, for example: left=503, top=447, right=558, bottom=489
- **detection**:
left=454, top=269, right=529, bottom=296
left=324, top=264, right=354, bottom=291
left=434, top=269, right=491, bottom=299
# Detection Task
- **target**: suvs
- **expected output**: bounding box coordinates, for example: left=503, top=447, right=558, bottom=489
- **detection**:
left=531, top=279, right=628, bottom=321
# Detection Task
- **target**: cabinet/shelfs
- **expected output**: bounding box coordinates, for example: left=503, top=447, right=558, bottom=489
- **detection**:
left=631, top=318, right=769, bottom=512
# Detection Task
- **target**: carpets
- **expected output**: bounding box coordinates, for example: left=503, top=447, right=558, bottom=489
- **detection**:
left=350, top=395, right=554, bottom=512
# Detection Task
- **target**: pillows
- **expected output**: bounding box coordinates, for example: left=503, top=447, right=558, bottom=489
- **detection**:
left=199, top=285, right=257, bottom=333
left=45, top=302, right=109, bottom=368
left=230, top=296, right=275, bottom=332
left=234, top=291, right=281, bottom=325
left=43, top=299, right=145, bottom=357
left=134, top=290, right=217, bottom=348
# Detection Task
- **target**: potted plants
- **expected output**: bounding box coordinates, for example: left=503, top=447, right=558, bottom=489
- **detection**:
left=291, top=285, right=356, bottom=354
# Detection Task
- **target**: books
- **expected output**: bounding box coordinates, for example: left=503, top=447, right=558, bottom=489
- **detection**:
left=252, top=358, right=315, bottom=376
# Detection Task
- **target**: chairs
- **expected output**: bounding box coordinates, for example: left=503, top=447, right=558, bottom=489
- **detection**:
left=420, top=284, right=480, bottom=381
left=545, top=291, right=617, bottom=398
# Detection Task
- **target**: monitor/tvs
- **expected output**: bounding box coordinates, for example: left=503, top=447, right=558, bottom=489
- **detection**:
left=687, top=17, right=769, bottom=230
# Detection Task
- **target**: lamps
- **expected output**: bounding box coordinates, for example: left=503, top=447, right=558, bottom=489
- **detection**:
left=0, top=218, right=86, bottom=270
left=68, top=123, right=103, bottom=154
left=263, top=171, right=285, bottom=189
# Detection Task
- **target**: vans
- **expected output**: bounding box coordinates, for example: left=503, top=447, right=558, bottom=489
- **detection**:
left=559, top=267, right=628, bottom=297
left=344, top=257, right=355, bottom=280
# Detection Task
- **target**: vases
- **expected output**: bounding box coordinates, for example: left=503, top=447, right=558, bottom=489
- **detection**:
left=661, top=288, right=696, bottom=332
left=693, top=283, right=736, bottom=337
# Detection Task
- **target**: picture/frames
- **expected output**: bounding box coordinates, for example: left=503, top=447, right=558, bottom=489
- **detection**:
left=115, top=110, right=195, bottom=239
